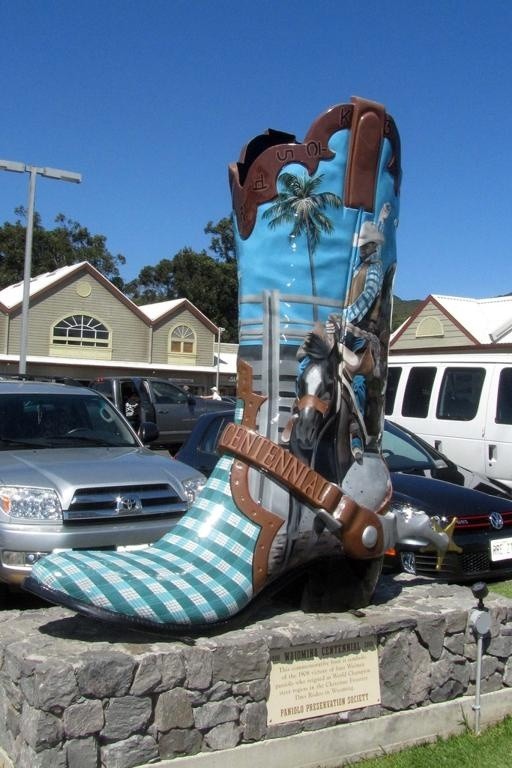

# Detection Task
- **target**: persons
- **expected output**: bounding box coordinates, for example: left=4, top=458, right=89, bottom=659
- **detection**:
left=178, top=385, right=223, bottom=401
left=296, top=221, right=385, bottom=461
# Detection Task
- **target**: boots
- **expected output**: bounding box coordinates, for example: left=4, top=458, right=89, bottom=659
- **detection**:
left=22, top=95, right=402, bottom=638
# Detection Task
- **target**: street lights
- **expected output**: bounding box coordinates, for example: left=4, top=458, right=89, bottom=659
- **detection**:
left=0, top=157, right=83, bottom=374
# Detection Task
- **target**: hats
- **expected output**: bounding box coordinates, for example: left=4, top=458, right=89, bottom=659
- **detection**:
left=353, top=220, right=385, bottom=247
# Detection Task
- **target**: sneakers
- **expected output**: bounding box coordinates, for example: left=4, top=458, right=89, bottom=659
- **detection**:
left=353, top=447, right=363, bottom=459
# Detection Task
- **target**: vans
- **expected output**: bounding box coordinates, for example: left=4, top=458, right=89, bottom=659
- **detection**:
left=381, top=351, right=512, bottom=491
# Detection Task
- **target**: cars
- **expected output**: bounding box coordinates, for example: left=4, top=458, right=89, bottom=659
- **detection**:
left=0, top=376, right=210, bottom=597
left=220, top=395, right=236, bottom=405
left=169, top=406, right=512, bottom=584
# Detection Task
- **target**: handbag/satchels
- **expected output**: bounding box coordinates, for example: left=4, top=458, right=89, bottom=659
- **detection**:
left=357, top=341, right=375, bottom=375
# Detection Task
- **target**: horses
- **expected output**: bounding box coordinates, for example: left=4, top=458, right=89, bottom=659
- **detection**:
left=282, top=261, right=397, bottom=567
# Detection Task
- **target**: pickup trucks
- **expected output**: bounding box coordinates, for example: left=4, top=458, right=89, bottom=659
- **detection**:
left=86, top=374, right=236, bottom=448
